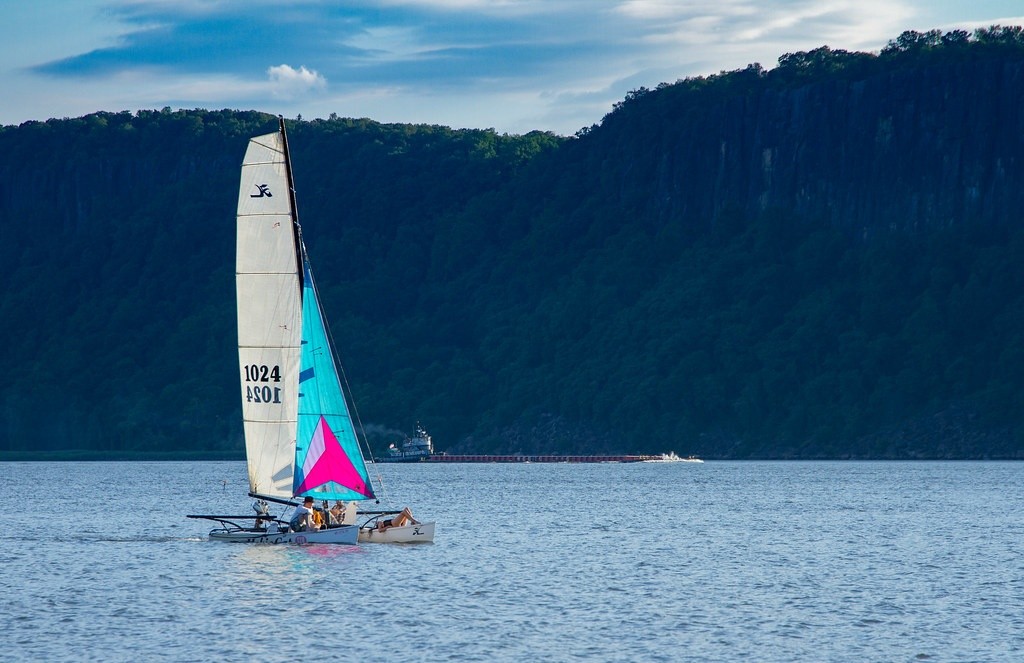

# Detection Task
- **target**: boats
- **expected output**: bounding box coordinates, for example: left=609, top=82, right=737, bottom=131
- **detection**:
left=642, top=451, right=704, bottom=463
left=372, top=420, right=434, bottom=463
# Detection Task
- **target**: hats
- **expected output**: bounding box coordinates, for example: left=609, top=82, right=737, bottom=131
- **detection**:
left=302, top=496, right=313, bottom=502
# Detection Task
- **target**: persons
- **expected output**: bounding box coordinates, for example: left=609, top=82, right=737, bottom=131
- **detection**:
left=330, top=500, right=346, bottom=525
left=368, top=506, right=421, bottom=539
left=290, top=496, right=327, bottom=533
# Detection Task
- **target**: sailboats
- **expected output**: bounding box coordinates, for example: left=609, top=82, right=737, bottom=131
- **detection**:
left=186, top=114, right=436, bottom=546
